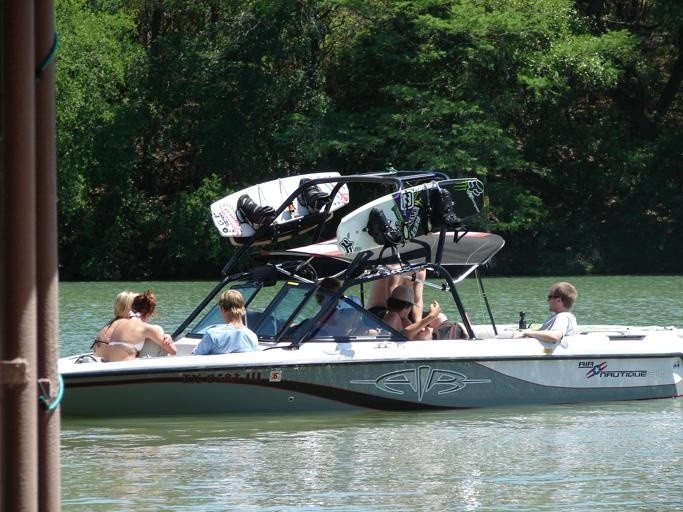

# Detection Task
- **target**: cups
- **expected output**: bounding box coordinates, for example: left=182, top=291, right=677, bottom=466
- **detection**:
left=549, top=295, right=559, bottom=299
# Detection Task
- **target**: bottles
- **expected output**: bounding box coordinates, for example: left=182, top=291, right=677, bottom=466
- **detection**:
left=391, top=285, right=419, bottom=308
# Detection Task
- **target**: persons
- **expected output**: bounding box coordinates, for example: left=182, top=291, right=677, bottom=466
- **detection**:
left=316, top=263, right=465, bottom=342
left=192, top=289, right=259, bottom=354
left=92, top=290, right=138, bottom=356
left=103, top=290, right=178, bottom=361
left=513, top=282, right=577, bottom=342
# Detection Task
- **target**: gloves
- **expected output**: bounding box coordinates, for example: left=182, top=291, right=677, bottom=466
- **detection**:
left=56, top=168, right=683, bottom=414
